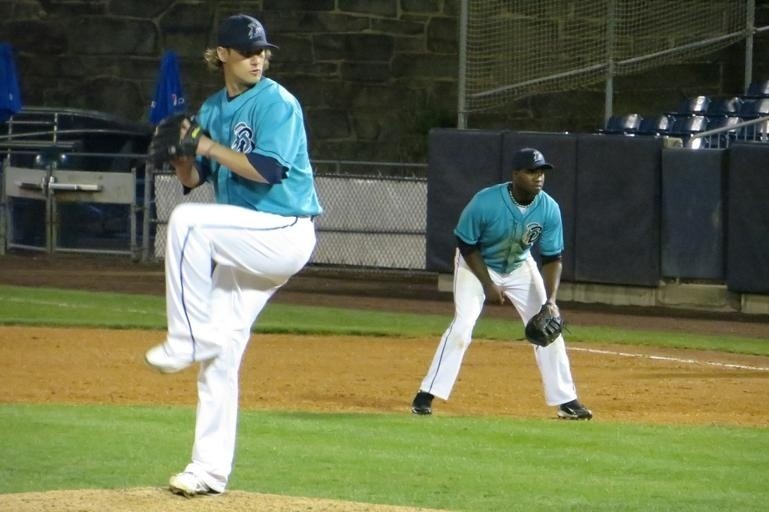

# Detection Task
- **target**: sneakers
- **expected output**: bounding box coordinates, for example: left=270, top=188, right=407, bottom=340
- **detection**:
left=559, top=404, right=592, bottom=420
left=144, top=341, right=187, bottom=371
left=170, top=475, right=210, bottom=498
left=411, top=393, right=432, bottom=415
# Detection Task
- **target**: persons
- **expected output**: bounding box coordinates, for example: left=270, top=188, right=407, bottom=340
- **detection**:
left=142, top=8, right=325, bottom=503
left=412, top=145, right=597, bottom=419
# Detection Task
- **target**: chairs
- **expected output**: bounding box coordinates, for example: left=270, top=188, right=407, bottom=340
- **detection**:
left=593, top=79, right=769, bottom=149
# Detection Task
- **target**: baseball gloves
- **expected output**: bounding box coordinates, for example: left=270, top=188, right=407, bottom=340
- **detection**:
left=147, top=113, right=210, bottom=170
left=525, top=303, right=562, bottom=346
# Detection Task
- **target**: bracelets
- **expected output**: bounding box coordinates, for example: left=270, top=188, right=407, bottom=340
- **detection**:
left=205, top=138, right=219, bottom=160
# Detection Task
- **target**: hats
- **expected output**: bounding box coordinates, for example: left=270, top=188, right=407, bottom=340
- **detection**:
left=220, top=15, right=279, bottom=52
left=511, top=148, right=554, bottom=171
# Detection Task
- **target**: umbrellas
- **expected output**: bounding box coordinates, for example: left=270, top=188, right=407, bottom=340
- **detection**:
left=146, top=45, right=186, bottom=129
left=0, top=41, right=24, bottom=129
